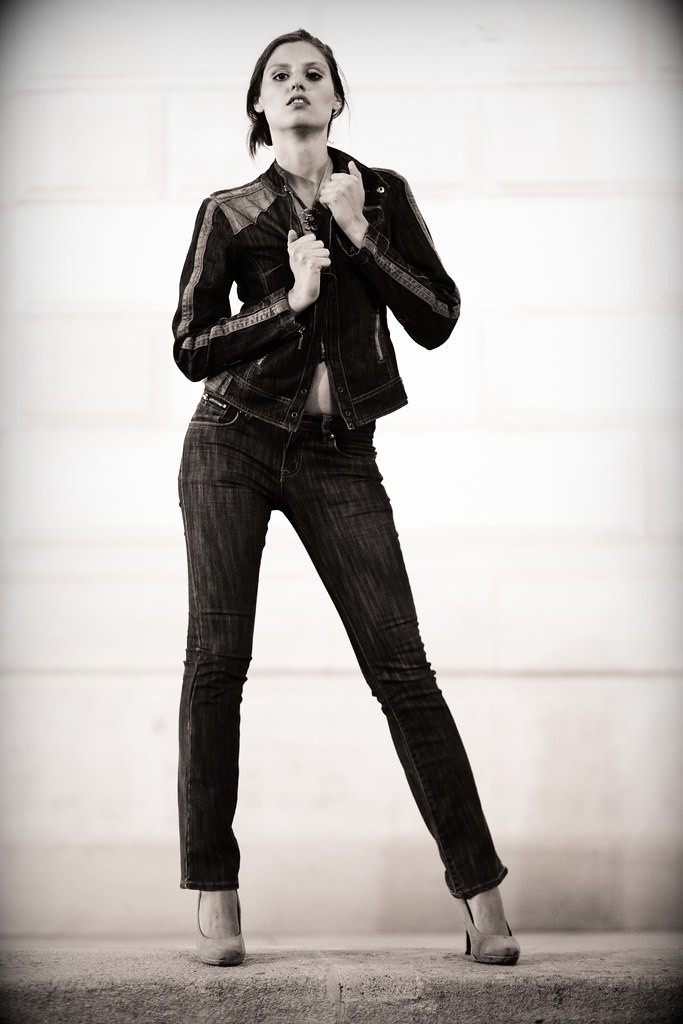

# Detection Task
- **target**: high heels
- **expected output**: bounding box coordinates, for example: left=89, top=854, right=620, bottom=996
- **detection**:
left=464, top=897, right=521, bottom=964
left=191, top=893, right=245, bottom=966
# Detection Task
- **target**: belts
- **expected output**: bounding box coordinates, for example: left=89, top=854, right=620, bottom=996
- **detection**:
left=299, top=410, right=355, bottom=459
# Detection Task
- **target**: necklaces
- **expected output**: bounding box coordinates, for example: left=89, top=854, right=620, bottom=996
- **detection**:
left=285, top=156, right=331, bottom=232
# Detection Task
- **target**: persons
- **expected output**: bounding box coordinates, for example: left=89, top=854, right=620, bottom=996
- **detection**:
left=173, top=28, right=522, bottom=967
